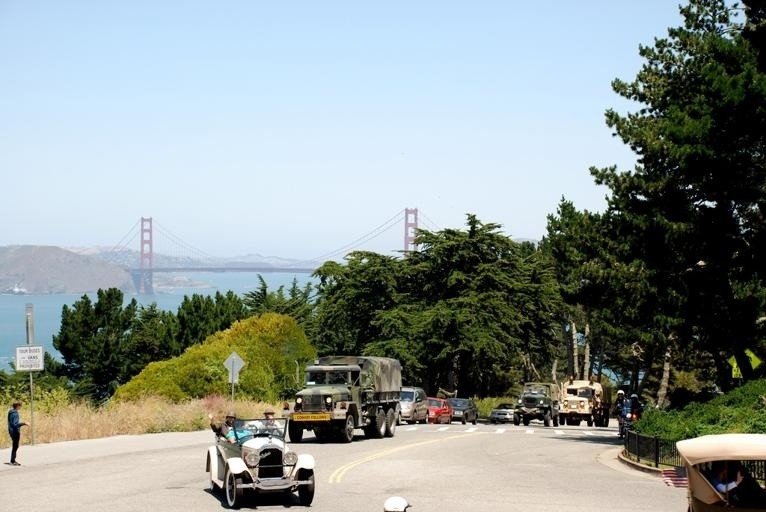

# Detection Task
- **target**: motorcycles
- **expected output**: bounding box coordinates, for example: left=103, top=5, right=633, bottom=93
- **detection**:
left=618, top=398, right=643, bottom=440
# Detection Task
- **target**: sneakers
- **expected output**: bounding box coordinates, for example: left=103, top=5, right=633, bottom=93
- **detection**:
left=10, top=461, right=21, bottom=466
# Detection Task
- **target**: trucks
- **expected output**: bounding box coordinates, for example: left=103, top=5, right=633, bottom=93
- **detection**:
left=288, top=356, right=402, bottom=444
left=513, top=381, right=560, bottom=427
left=558, top=376, right=604, bottom=427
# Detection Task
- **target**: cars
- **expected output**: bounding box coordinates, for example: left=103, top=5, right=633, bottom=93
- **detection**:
left=397, top=385, right=523, bottom=426
left=206, top=418, right=316, bottom=510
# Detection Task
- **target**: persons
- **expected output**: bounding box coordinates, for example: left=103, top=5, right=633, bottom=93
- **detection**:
left=615, top=389, right=630, bottom=419
left=629, top=393, right=645, bottom=418
left=216, top=409, right=236, bottom=438
left=7, top=400, right=31, bottom=466
left=226, top=418, right=252, bottom=445
left=259, top=408, right=283, bottom=437
left=709, top=461, right=746, bottom=507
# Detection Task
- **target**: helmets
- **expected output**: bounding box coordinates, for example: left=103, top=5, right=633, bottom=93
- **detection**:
left=263, top=408, right=275, bottom=415
left=226, top=411, right=236, bottom=419
left=616, top=390, right=638, bottom=399
